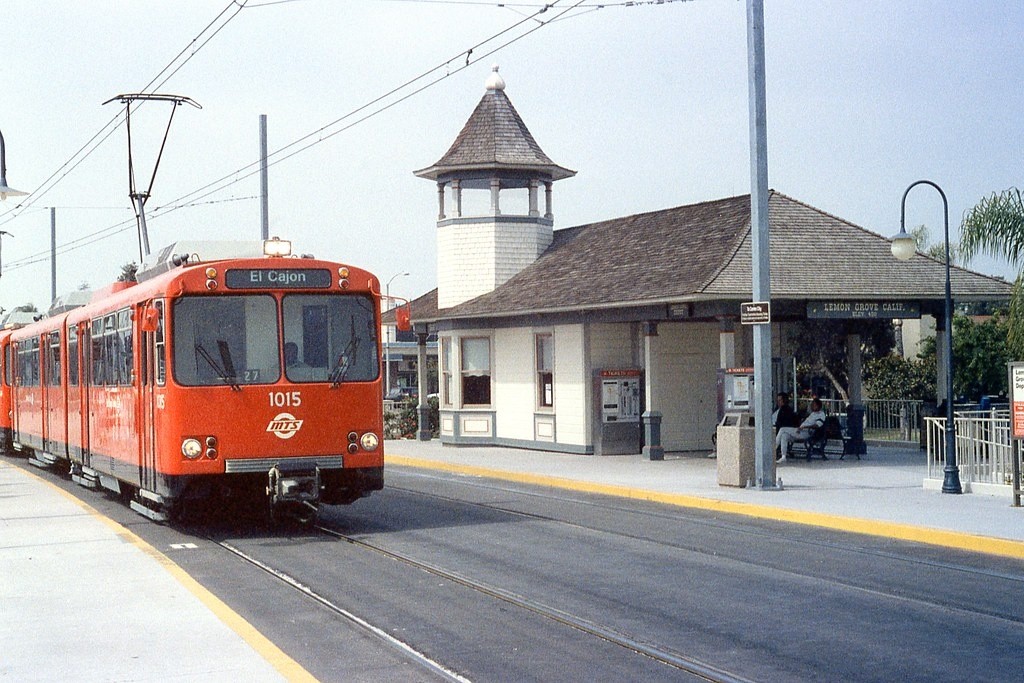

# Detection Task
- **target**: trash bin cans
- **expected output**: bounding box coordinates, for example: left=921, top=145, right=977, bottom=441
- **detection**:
left=716, top=413, right=776, bottom=487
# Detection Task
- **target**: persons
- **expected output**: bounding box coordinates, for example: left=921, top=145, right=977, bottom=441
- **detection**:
left=272, top=344, right=311, bottom=371
left=775, top=398, right=824, bottom=465
left=772, top=394, right=795, bottom=434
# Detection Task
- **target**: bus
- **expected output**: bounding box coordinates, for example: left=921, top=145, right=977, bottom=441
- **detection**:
left=0, top=232, right=412, bottom=538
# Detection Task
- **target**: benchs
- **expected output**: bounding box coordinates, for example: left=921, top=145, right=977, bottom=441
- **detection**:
left=788, top=415, right=861, bottom=463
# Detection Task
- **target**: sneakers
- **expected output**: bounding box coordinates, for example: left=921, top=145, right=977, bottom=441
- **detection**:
left=776, top=458, right=787, bottom=464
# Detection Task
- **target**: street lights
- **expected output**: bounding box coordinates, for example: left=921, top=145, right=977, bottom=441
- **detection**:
left=384, top=272, right=411, bottom=396
left=885, top=180, right=964, bottom=495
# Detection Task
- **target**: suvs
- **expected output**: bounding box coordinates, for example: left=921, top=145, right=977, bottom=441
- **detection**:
left=384, top=386, right=419, bottom=409
